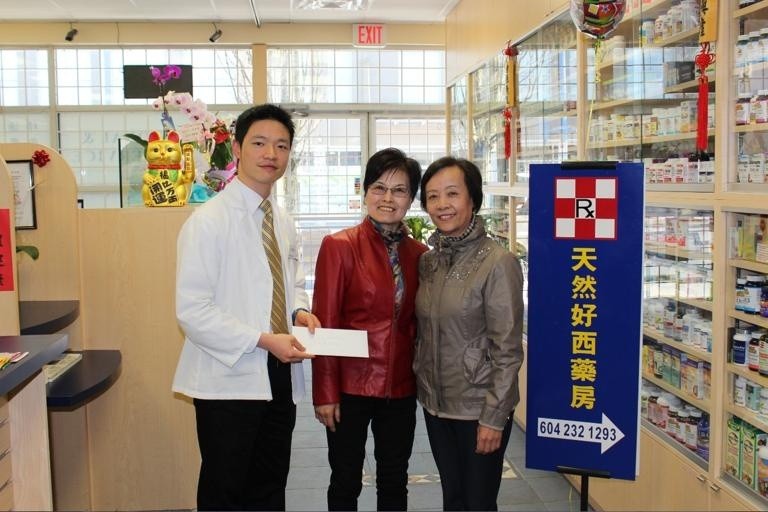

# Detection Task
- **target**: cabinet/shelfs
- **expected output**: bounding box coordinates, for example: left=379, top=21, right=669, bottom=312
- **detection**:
left=443, top=0, right=767, bottom=509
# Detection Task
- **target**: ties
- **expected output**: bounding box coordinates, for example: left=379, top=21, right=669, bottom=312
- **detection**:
left=259, top=199, right=289, bottom=334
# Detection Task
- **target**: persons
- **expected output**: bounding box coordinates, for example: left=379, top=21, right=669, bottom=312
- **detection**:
left=171, top=105, right=524, bottom=511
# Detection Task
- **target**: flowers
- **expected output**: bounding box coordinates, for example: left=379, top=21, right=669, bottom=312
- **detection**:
left=149, top=63, right=237, bottom=192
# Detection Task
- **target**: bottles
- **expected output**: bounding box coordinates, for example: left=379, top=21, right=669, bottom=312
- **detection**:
left=638, top=2, right=697, bottom=49
left=735, top=90, right=768, bottom=125
left=733, top=327, right=768, bottom=377
left=736, top=29, right=768, bottom=94
left=599, top=34, right=627, bottom=67
left=734, top=276, right=768, bottom=318
left=638, top=386, right=709, bottom=461
left=642, top=299, right=714, bottom=353
left=589, top=114, right=684, bottom=143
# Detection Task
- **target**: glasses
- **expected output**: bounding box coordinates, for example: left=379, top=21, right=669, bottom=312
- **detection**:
left=368, top=185, right=409, bottom=198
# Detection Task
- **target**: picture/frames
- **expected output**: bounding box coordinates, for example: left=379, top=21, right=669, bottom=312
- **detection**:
left=6, top=158, right=37, bottom=232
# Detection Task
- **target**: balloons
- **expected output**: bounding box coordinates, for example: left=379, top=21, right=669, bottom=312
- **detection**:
left=570, top=0, right=628, bottom=40
